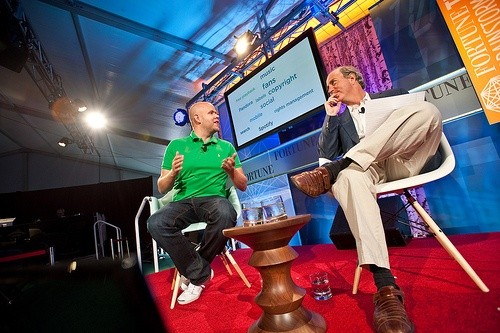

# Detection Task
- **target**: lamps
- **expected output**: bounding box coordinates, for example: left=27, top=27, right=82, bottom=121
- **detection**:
left=234, top=29, right=258, bottom=56
left=58, top=137, right=70, bottom=148
left=0, top=39, right=32, bottom=73
left=74, top=98, right=87, bottom=112
left=173, top=108, right=190, bottom=126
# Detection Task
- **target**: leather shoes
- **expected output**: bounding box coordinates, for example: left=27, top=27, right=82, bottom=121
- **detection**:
left=290, top=166, right=332, bottom=198
left=372, top=286, right=414, bottom=333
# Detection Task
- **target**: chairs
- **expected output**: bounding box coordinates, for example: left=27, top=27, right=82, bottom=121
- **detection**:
left=351, top=132, right=490, bottom=294
left=170, top=186, right=251, bottom=308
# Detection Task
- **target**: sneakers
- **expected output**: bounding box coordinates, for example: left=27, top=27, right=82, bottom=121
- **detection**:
left=180, top=276, right=190, bottom=289
left=177, top=268, right=214, bottom=305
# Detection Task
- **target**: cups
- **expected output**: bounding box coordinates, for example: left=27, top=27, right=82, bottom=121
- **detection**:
left=242, top=203, right=264, bottom=227
left=260, top=195, right=287, bottom=224
left=309, top=272, right=332, bottom=301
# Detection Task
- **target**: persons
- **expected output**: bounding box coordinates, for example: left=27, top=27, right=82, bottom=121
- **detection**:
left=147, top=102, right=247, bottom=305
left=291, top=65, right=443, bottom=333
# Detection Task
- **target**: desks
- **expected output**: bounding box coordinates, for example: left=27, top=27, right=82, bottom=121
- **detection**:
left=222, top=214, right=327, bottom=333
